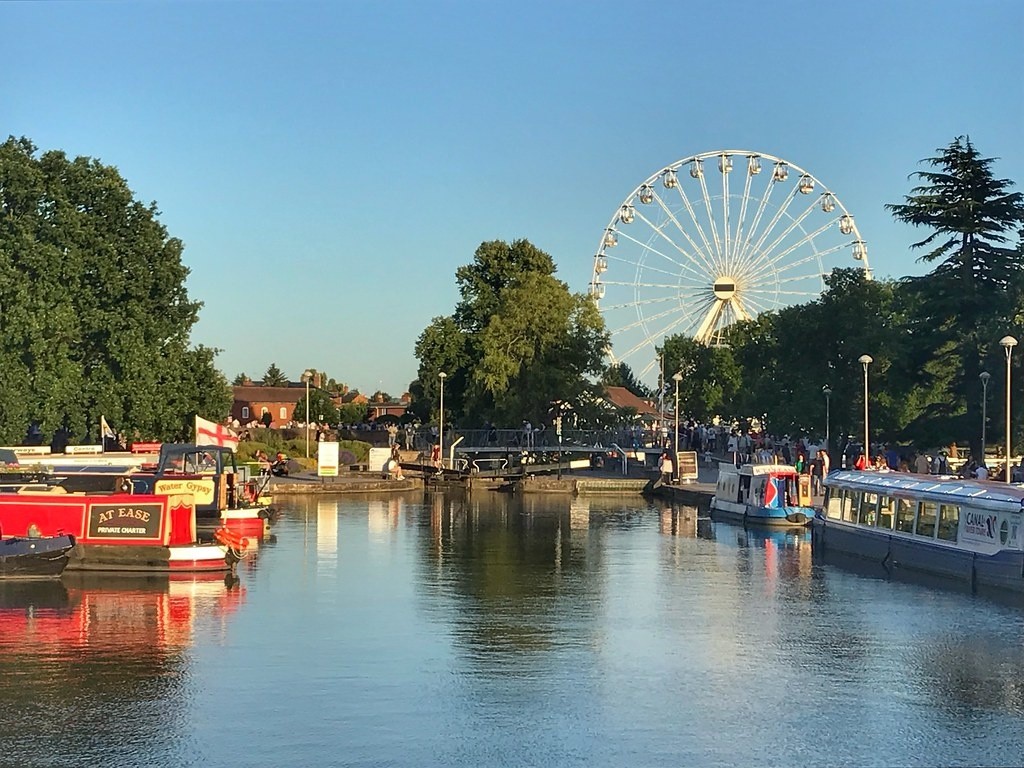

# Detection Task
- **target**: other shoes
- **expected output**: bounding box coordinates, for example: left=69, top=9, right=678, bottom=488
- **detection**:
left=400, top=475, right=405, bottom=479
left=397, top=477, right=403, bottom=480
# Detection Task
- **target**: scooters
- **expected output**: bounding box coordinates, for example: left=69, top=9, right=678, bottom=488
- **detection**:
left=272, top=459, right=288, bottom=477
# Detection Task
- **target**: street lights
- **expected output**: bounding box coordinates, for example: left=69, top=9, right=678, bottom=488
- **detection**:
left=671, top=374, right=683, bottom=456
left=858, top=355, right=873, bottom=470
left=999, top=336, right=1017, bottom=485
left=822, top=388, right=832, bottom=440
left=979, top=371, right=991, bottom=468
left=305, top=371, right=312, bottom=458
left=439, top=372, right=446, bottom=469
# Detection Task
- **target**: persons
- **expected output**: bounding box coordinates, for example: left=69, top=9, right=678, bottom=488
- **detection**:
left=238, top=410, right=1024, bottom=496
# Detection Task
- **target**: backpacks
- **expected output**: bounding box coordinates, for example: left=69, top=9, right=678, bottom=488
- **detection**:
left=938, top=457, right=946, bottom=474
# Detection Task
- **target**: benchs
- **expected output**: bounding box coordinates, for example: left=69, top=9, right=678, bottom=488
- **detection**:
left=383, top=472, right=396, bottom=481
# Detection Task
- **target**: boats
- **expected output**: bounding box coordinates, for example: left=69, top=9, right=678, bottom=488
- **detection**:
left=709, top=450, right=816, bottom=526
left=0, top=443, right=277, bottom=582
left=812, top=469, right=1024, bottom=593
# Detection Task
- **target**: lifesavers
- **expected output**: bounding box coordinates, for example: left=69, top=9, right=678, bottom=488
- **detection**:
left=215, top=529, right=250, bottom=552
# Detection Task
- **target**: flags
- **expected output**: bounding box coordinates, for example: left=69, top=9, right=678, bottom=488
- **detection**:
left=99, top=415, right=116, bottom=440
left=195, top=414, right=238, bottom=454
